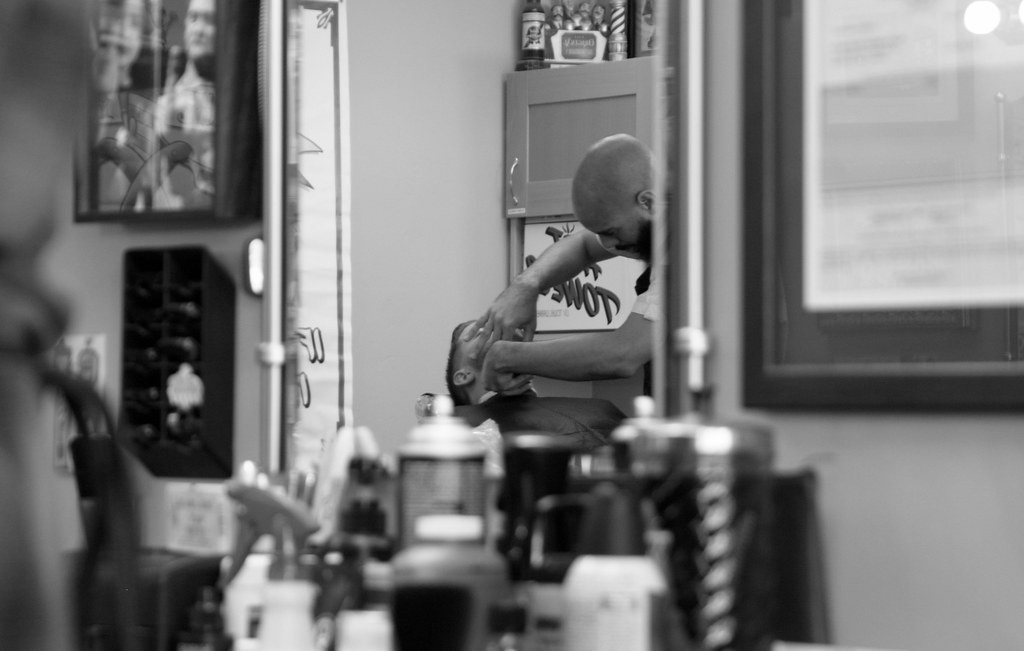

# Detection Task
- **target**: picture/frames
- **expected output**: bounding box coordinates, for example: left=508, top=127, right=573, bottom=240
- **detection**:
left=71, top=0, right=258, bottom=228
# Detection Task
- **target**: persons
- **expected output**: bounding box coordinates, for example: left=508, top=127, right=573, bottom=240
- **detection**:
left=444, top=134, right=652, bottom=406
left=91, top=1, right=219, bottom=209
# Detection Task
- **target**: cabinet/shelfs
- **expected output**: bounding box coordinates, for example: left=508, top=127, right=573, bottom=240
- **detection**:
left=116, top=245, right=237, bottom=480
left=501, top=53, right=655, bottom=421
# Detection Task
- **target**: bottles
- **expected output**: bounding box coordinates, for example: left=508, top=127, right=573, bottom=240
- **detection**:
left=390, top=515, right=511, bottom=651
left=396, top=392, right=489, bottom=553
left=611, top=422, right=773, bottom=650
left=520, top=0, right=544, bottom=61
